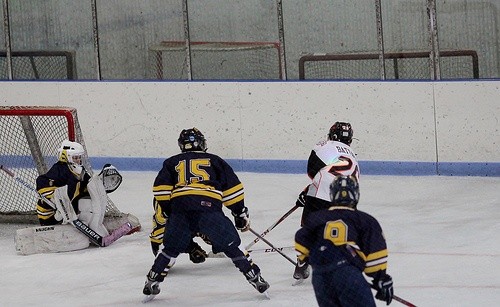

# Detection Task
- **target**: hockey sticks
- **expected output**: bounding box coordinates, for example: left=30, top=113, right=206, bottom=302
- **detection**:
left=245, top=205, right=299, bottom=251
left=0, top=165, right=133, bottom=247
left=370, top=284, right=416, bottom=307
left=208, top=246, right=295, bottom=259
left=249, top=227, right=310, bottom=279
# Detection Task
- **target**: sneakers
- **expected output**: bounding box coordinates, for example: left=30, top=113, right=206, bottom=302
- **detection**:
left=292, top=255, right=310, bottom=286
left=143, top=275, right=160, bottom=304
left=249, top=272, right=270, bottom=298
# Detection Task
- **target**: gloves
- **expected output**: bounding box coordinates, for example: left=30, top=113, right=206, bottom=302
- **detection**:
left=296, top=191, right=307, bottom=207
left=231, top=206, right=250, bottom=232
left=190, top=242, right=208, bottom=263
left=372, top=274, right=394, bottom=305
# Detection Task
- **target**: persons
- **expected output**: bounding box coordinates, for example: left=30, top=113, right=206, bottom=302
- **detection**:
left=16, top=140, right=141, bottom=255
left=143, top=126, right=271, bottom=302
left=294, top=122, right=361, bottom=279
left=295, top=175, right=394, bottom=307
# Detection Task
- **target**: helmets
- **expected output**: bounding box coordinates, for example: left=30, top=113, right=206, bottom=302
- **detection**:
left=330, top=175, right=360, bottom=207
left=58, top=139, right=88, bottom=175
left=330, top=121, right=354, bottom=146
left=178, top=127, right=208, bottom=152
